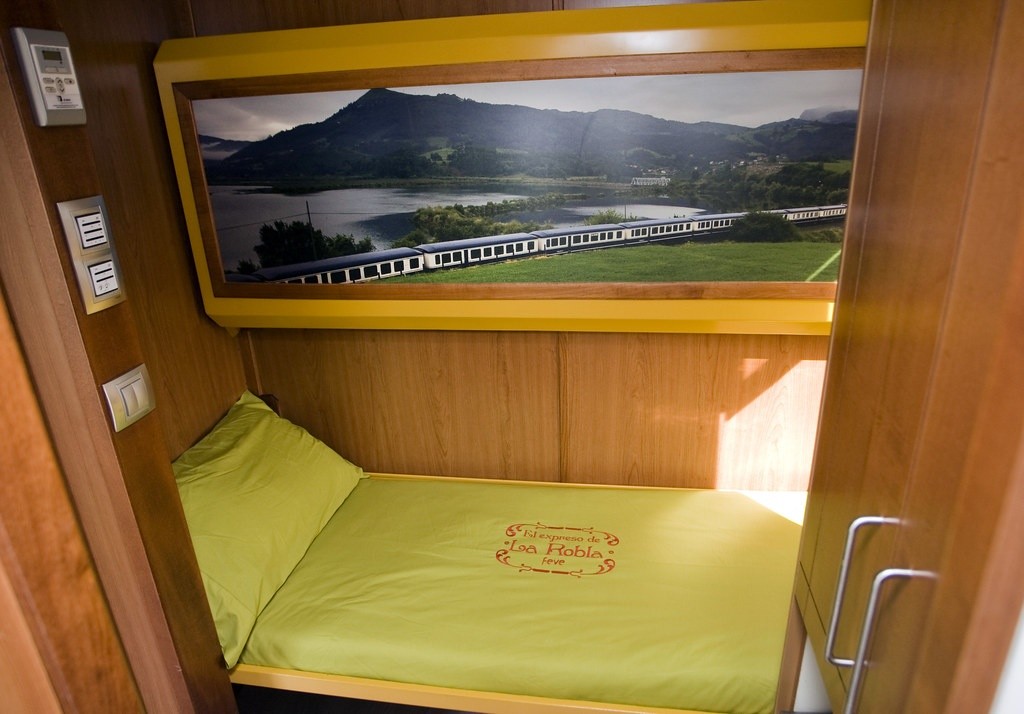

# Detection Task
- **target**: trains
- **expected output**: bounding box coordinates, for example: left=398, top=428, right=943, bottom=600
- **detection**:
left=225, top=204, right=847, bottom=285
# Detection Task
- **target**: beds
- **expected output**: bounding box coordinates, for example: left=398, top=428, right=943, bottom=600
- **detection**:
left=170, top=390, right=809, bottom=714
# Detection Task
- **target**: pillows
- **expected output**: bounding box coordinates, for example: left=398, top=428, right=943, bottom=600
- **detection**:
left=170, top=391, right=369, bottom=670
